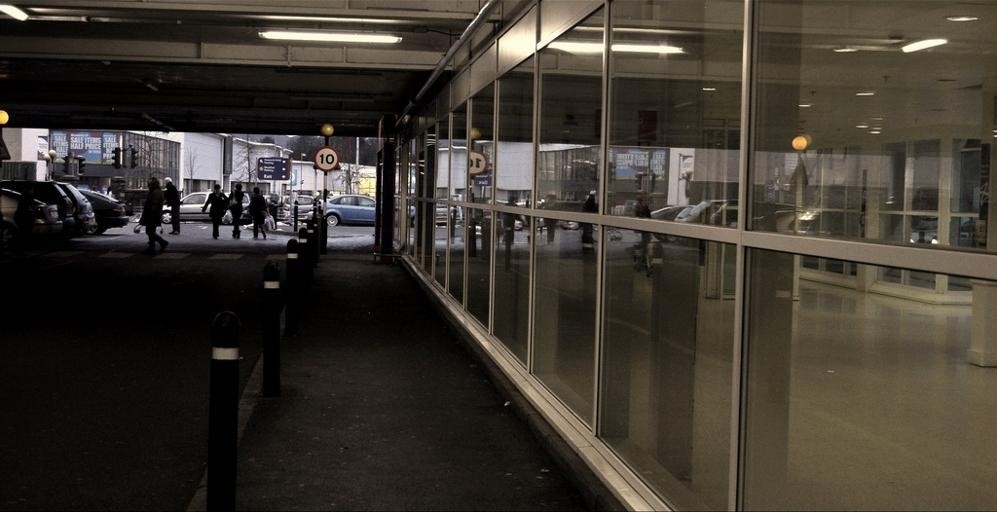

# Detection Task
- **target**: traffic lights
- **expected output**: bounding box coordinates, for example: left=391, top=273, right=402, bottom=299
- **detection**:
left=588, top=162, right=597, bottom=183
left=111, top=147, right=121, bottom=170
left=76, top=155, right=85, bottom=175
left=62, top=156, right=70, bottom=174
left=608, top=162, right=616, bottom=183
left=131, top=148, right=139, bottom=169
left=651, top=172, right=660, bottom=190
left=634, top=172, right=642, bottom=189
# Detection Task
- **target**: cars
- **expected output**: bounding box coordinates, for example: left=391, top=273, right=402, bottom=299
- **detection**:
left=394, top=195, right=462, bottom=226
left=162, top=192, right=314, bottom=225
left=319, top=194, right=376, bottom=226
left=485, top=198, right=584, bottom=230
left=0, top=180, right=128, bottom=251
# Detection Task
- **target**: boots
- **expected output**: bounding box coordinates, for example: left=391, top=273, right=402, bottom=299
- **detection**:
left=231, top=230, right=241, bottom=238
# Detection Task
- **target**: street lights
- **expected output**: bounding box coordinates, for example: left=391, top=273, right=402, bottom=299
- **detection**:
left=792, top=134, right=807, bottom=297
left=0, top=111, right=8, bottom=141
left=468, top=127, right=480, bottom=226
left=323, top=125, right=334, bottom=216
left=45, top=150, right=56, bottom=180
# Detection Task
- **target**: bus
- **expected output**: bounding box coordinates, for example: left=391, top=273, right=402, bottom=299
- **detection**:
left=125, top=188, right=183, bottom=214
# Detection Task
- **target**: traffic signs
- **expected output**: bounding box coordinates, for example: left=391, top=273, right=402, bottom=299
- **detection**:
left=257, top=156, right=291, bottom=180
left=316, top=148, right=337, bottom=171
left=473, top=164, right=493, bottom=185
left=470, top=152, right=486, bottom=174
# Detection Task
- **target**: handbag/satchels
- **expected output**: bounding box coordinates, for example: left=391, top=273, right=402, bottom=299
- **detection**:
left=229, top=197, right=238, bottom=208
left=246, top=207, right=253, bottom=216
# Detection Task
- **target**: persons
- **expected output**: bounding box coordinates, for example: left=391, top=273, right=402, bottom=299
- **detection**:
left=262, top=192, right=281, bottom=231
left=163, top=176, right=180, bottom=235
left=503, top=195, right=525, bottom=246
left=108, top=187, right=112, bottom=198
left=249, top=187, right=267, bottom=240
left=140, top=176, right=168, bottom=253
left=634, top=189, right=656, bottom=277
left=575, top=192, right=597, bottom=249
left=202, top=184, right=228, bottom=239
left=229, top=184, right=244, bottom=239
left=542, top=192, right=560, bottom=243
